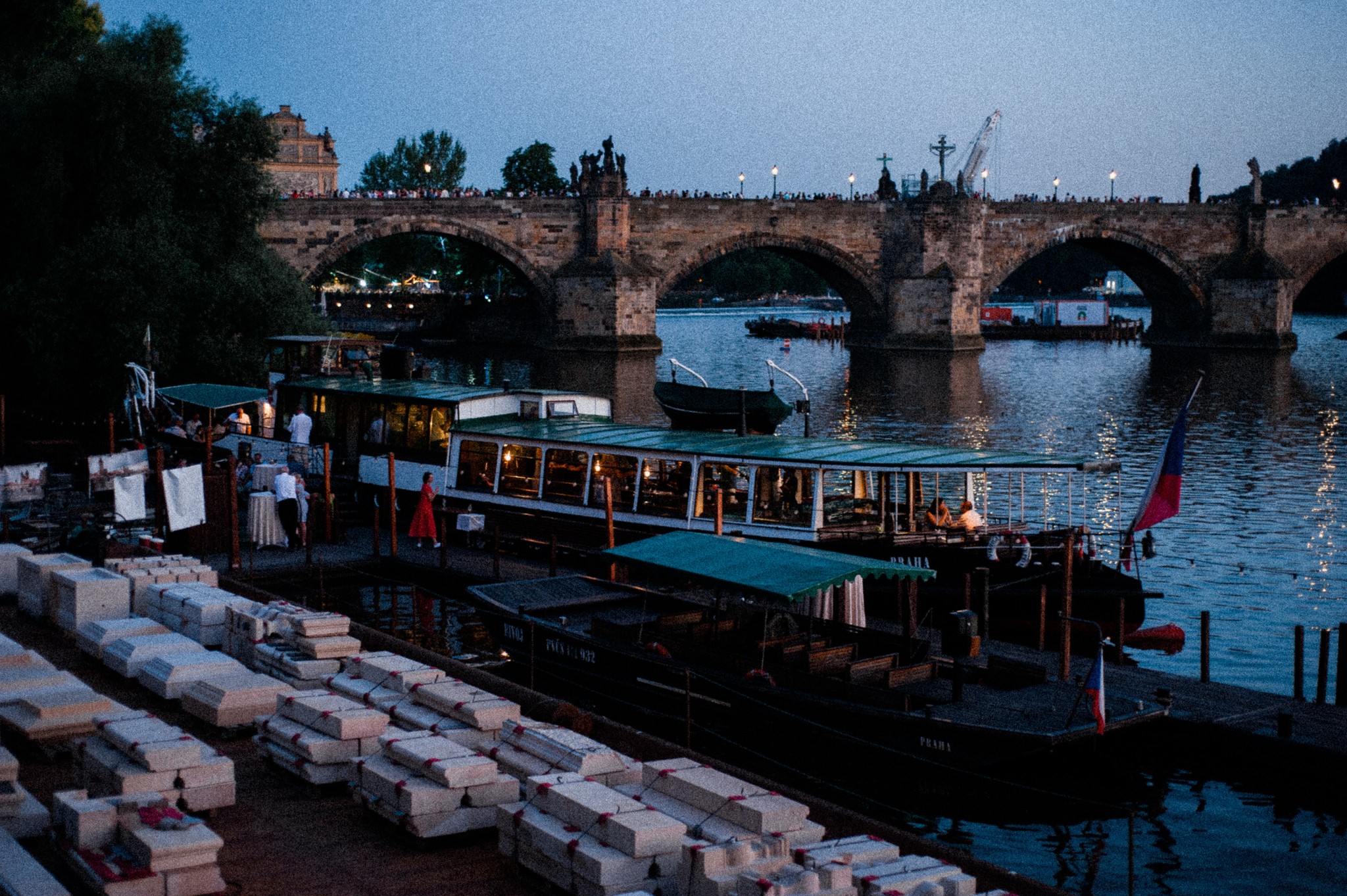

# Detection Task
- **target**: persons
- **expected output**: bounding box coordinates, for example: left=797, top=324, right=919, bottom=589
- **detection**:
left=951, top=501, right=982, bottom=542
left=669, top=460, right=741, bottom=504
left=186, top=414, right=202, bottom=436
left=771, top=465, right=798, bottom=520
left=134, top=439, right=145, bottom=450
left=193, top=425, right=206, bottom=443
left=225, top=407, right=251, bottom=435
left=275, top=454, right=310, bottom=552
left=236, top=453, right=268, bottom=499
left=165, top=417, right=187, bottom=439
left=288, top=405, right=313, bottom=478
left=409, top=472, right=441, bottom=548
left=924, top=497, right=951, bottom=531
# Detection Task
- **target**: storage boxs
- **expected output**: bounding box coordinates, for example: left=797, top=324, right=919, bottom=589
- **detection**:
left=151, top=538, right=164, bottom=553
left=139, top=534, right=152, bottom=547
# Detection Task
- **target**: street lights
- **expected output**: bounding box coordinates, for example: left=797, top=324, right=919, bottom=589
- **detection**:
left=737, top=172, right=746, bottom=197
left=1052, top=176, right=1060, bottom=202
left=847, top=172, right=856, bottom=202
left=980, top=166, right=990, bottom=201
left=770, top=164, right=779, bottom=200
left=1109, top=168, right=1118, bottom=201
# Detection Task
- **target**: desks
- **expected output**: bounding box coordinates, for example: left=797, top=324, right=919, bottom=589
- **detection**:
left=252, top=464, right=286, bottom=491
left=248, top=492, right=289, bottom=549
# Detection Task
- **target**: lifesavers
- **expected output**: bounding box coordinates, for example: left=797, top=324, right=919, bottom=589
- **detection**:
left=743, top=668, right=778, bottom=690
left=1076, top=525, right=1097, bottom=561
left=644, top=642, right=671, bottom=660
left=818, top=317, right=825, bottom=324
left=987, top=530, right=1032, bottom=569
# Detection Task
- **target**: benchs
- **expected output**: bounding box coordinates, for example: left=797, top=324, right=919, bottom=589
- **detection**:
left=658, top=611, right=935, bottom=696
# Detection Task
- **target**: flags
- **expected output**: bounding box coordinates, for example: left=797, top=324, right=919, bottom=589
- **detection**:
left=1085, top=650, right=1110, bottom=732
left=1134, top=408, right=1186, bottom=531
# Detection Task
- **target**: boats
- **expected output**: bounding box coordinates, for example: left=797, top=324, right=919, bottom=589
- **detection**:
left=743, top=298, right=1148, bottom=343
left=110, top=359, right=1178, bottom=829
left=653, top=356, right=820, bottom=441
left=263, top=333, right=432, bottom=381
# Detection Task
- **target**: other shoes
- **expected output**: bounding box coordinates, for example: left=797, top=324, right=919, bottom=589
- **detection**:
left=417, top=543, right=422, bottom=547
left=434, top=543, right=440, bottom=548
